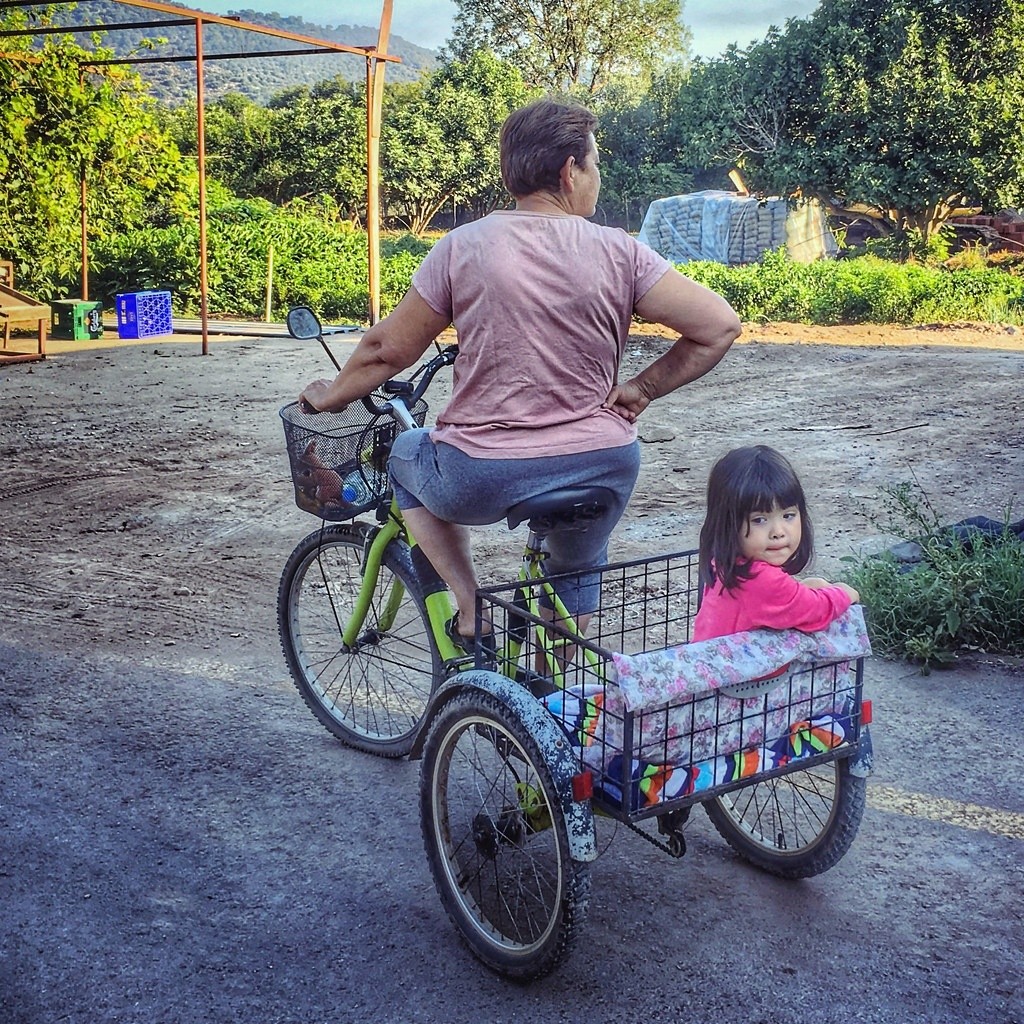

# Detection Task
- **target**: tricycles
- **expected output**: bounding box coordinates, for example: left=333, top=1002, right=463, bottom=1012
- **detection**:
left=269, top=304, right=878, bottom=979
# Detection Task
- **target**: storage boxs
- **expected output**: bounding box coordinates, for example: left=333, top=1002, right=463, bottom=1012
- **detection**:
left=543, top=668, right=848, bottom=808
left=116, top=291, right=173, bottom=339
left=51, top=297, right=102, bottom=340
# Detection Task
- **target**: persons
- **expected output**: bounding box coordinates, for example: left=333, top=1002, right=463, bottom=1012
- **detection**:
left=690, top=445, right=860, bottom=644
left=298, top=99, right=741, bottom=682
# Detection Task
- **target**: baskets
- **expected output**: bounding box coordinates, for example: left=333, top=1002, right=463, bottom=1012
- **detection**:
left=278, top=384, right=428, bottom=523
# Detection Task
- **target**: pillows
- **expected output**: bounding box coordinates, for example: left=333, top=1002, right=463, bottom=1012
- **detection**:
left=596, top=612, right=873, bottom=760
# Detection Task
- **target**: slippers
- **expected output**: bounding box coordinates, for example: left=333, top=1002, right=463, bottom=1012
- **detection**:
left=445, top=610, right=495, bottom=654
